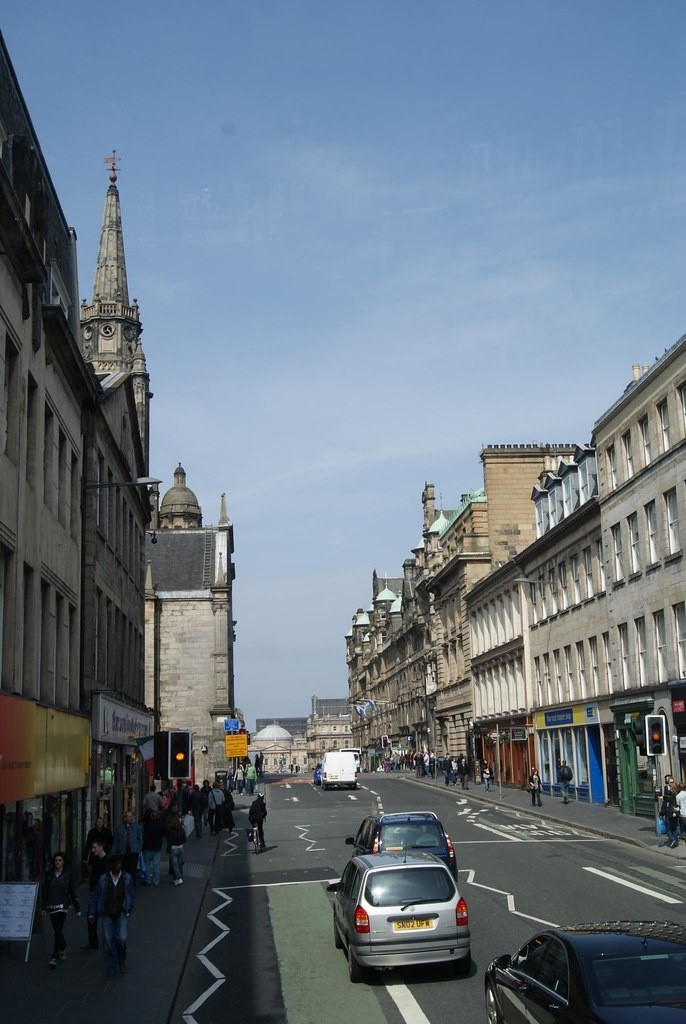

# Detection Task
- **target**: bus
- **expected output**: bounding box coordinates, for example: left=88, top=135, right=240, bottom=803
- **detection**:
left=339, top=748, right=362, bottom=773
left=382, top=735, right=388, bottom=748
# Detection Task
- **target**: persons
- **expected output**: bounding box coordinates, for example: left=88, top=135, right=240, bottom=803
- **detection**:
left=459, top=759, right=471, bottom=790
left=101, top=764, right=112, bottom=784
left=255, top=751, right=264, bottom=777
left=183, top=780, right=235, bottom=838
left=165, top=799, right=185, bottom=886
left=481, top=759, right=495, bottom=793
left=558, top=760, right=573, bottom=804
left=442, top=753, right=459, bottom=787
left=248, top=792, right=267, bottom=847
left=88, top=855, right=135, bottom=977
left=23, top=811, right=52, bottom=875
left=392, top=750, right=435, bottom=779
left=40, top=851, right=82, bottom=968
left=235, top=761, right=256, bottom=796
left=80, top=839, right=115, bottom=950
left=112, top=811, right=143, bottom=887
left=87, top=816, right=112, bottom=851
left=659, top=774, right=686, bottom=849
left=144, top=784, right=170, bottom=816
left=529, top=769, right=543, bottom=806
left=139, top=808, right=162, bottom=886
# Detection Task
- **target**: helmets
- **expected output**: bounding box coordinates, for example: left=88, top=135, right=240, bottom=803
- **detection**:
left=257, top=792, right=264, bottom=798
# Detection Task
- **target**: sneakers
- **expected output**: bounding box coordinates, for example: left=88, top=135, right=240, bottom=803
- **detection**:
left=174, top=878, right=183, bottom=885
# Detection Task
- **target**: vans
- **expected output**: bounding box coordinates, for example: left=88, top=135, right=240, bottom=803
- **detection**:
left=320, top=751, right=358, bottom=790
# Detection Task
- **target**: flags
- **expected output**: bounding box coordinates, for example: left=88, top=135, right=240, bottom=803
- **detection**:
left=355, top=700, right=378, bottom=716
left=136, top=736, right=154, bottom=776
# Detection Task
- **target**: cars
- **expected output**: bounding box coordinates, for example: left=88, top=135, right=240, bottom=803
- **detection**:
left=345, top=811, right=459, bottom=884
left=326, top=851, right=471, bottom=984
left=484, top=920, right=686, bottom=1024
left=312, top=761, right=323, bottom=785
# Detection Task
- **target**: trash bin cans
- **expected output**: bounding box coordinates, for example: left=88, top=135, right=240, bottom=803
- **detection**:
left=214, top=771, right=228, bottom=788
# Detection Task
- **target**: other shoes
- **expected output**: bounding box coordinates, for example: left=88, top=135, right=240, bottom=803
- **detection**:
left=120, top=965, right=127, bottom=974
left=154, top=880, right=159, bottom=885
left=143, top=879, right=151, bottom=885
left=49, top=955, right=58, bottom=968
left=81, top=939, right=98, bottom=950
left=106, top=971, right=115, bottom=976
left=260, top=842, right=266, bottom=848
left=60, top=950, right=66, bottom=960
left=670, top=840, right=676, bottom=848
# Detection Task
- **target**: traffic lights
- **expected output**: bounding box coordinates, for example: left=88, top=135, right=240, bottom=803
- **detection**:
left=645, top=714, right=667, bottom=756
left=168, top=730, right=191, bottom=779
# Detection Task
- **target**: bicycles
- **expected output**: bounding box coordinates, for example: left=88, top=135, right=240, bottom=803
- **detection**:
left=252, top=816, right=267, bottom=854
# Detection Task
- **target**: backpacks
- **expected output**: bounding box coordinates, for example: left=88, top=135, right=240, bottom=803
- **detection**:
left=561, top=767, right=568, bottom=779
left=669, top=802, right=680, bottom=819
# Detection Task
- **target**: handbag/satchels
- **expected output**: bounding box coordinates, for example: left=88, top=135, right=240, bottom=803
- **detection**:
left=657, top=817, right=667, bottom=834
left=139, top=851, right=146, bottom=871
left=483, top=773, right=490, bottom=778
left=215, top=804, right=224, bottom=815
